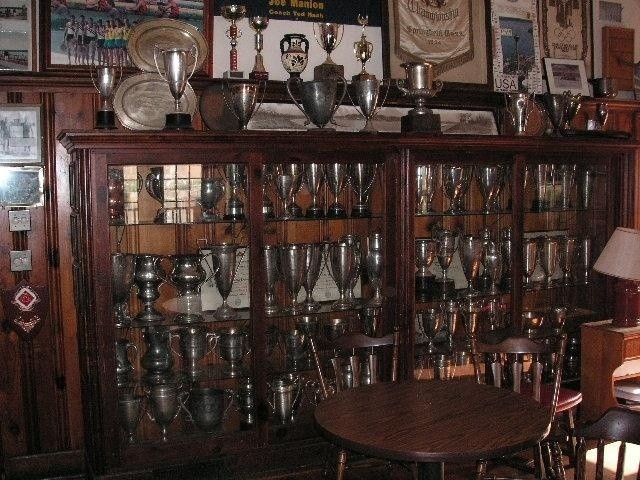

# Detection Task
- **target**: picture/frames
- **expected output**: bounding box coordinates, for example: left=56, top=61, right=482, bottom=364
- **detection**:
left=0, top=101, right=45, bottom=168
left=0, top=0, right=214, bottom=82
left=490, top=6, right=542, bottom=95
left=542, top=56, right=590, bottom=98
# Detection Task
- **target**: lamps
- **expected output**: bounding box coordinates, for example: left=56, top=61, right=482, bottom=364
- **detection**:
left=592, top=227, right=640, bottom=328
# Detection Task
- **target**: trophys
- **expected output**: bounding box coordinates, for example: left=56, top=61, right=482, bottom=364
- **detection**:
left=221, top=78, right=267, bottom=131
left=396, top=60, right=445, bottom=135
left=287, top=72, right=347, bottom=132
left=248, top=14, right=270, bottom=80
left=152, top=45, right=195, bottom=132
left=504, top=89, right=538, bottom=135
left=558, top=88, right=583, bottom=136
left=311, top=20, right=345, bottom=79
left=90, top=60, right=123, bottom=129
left=344, top=70, right=391, bottom=134
left=220, top=3, right=246, bottom=79
left=352, top=13, right=377, bottom=82
left=596, top=101, right=608, bottom=131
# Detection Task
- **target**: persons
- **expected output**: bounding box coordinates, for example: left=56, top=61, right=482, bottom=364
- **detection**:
left=50, top=0, right=181, bottom=70
left=0, top=117, right=13, bottom=155
left=21, top=118, right=35, bottom=153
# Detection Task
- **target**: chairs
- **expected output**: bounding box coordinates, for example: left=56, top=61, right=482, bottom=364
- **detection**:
left=306, top=326, right=419, bottom=479
left=468, top=307, right=640, bottom=479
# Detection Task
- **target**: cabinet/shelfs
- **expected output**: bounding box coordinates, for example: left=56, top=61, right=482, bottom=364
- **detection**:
left=57, top=129, right=640, bottom=479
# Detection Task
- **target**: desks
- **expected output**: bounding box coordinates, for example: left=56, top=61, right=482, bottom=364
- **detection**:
left=311, top=380, right=551, bottom=479
